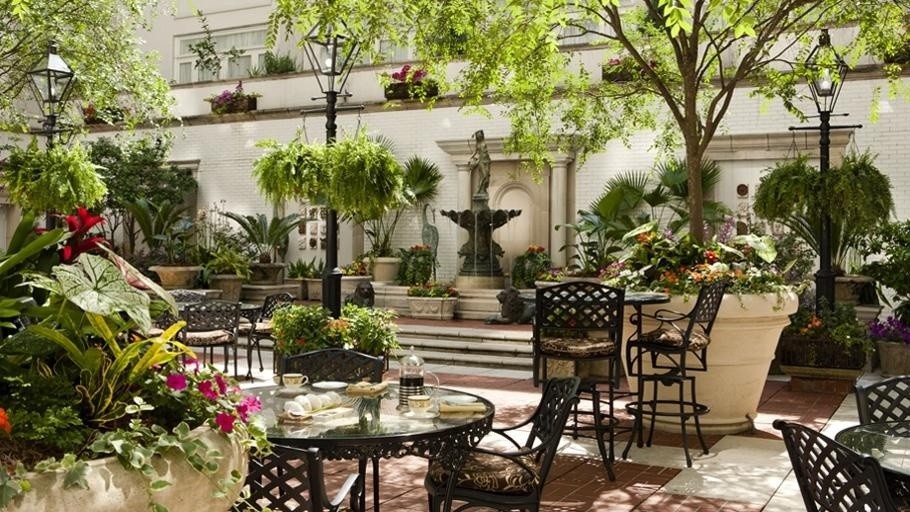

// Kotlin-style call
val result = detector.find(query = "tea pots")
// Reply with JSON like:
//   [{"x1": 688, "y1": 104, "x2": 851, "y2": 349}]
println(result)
[{"x1": 396, "y1": 346, "x2": 439, "y2": 410}]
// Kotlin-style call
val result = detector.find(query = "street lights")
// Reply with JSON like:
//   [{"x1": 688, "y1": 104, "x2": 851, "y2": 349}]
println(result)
[
  {"x1": 803, "y1": 21, "x2": 851, "y2": 310},
  {"x1": 304, "y1": 14, "x2": 362, "y2": 316},
  {"x1": 26, "y1": 39, "x2": 73, "y2": 234}
]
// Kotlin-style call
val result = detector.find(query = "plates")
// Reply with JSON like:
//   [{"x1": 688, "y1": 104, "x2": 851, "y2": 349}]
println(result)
[
  {"x1": 277, "y1": 389, "x2": 310, "y2": 395},
  {"x1": 401, "y1": 411, "x2": 441, "y2": 419},
  {"x1": 441, "y1": 395, "x2": 478, "y2": 403},
  {"x1": 313, "y1": 381, "x2": 348, "y2": 389}
]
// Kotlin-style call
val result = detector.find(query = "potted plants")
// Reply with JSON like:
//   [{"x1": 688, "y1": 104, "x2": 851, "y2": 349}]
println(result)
[
  {"x1": 756, "y1": 147, "x2": 910, "y2": 308},
  {"x1": 345, "y1": 136, "x2": 443, "y2": 282},
  {"x1": 117, "y1": 195, "x2": 327, "y2": 302}
]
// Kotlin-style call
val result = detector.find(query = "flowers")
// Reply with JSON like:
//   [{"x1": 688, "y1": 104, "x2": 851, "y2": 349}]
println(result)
[
  {"x1": 380, "y1": 64, "x2": 432, "y2": 82},
  {"x1": 606, "y1": 56, "x2": 659, "y2": 70},
  {"x1": 82, "y1": 101, "x2": 132, "y2": 116},
  {"x1": 867, "y1": 317, "x2": 910, "y2": 344},
  {"x1": 780, "y1": 297, "x2": 864, "y2": 351},
  {"x1": 204, "y1": 80, "x2": 263, "y2": 114}
]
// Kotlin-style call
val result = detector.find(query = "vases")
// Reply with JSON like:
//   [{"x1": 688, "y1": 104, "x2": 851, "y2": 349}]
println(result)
[
  {"x1": 874, "y1": 340, "x2": 910, "y2": 377},
  {"x1": 210, "y1": 97, "x2": 258, "y2": 112},
  {"x1": 782, "y1": 332, "x2": 865, "y2": 370},
  {"x1": 601, "y1": 68, "x2": 657, "y2": 83},
  {"x1": 84, "y1": 113, "x2": 123, "y2": 124},
  {"x1": 384, "y1": 81, "x2": 438, "y2": 99}
]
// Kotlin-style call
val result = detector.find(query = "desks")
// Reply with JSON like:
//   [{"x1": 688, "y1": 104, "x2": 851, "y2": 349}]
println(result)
[{"x1": 835, "y1": 420, "x2": 910, "y2": 512}]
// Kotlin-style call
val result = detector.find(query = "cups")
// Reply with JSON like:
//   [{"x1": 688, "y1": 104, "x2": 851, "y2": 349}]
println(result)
[
  {"x1": 408, "y1": 396, "x2": 434, "y2": 415},
  {"x1": 283, "y1": 374, "x2": 308, "y2": 389}
]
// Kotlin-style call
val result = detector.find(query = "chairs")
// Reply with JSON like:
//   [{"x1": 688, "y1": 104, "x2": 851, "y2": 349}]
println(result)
[
  {"x1": 855, "y1": 375, "x2": 909, "y2": 436},
  {"x1": 773, "y1": 420, "x2": 898, "y2": 511}
]
